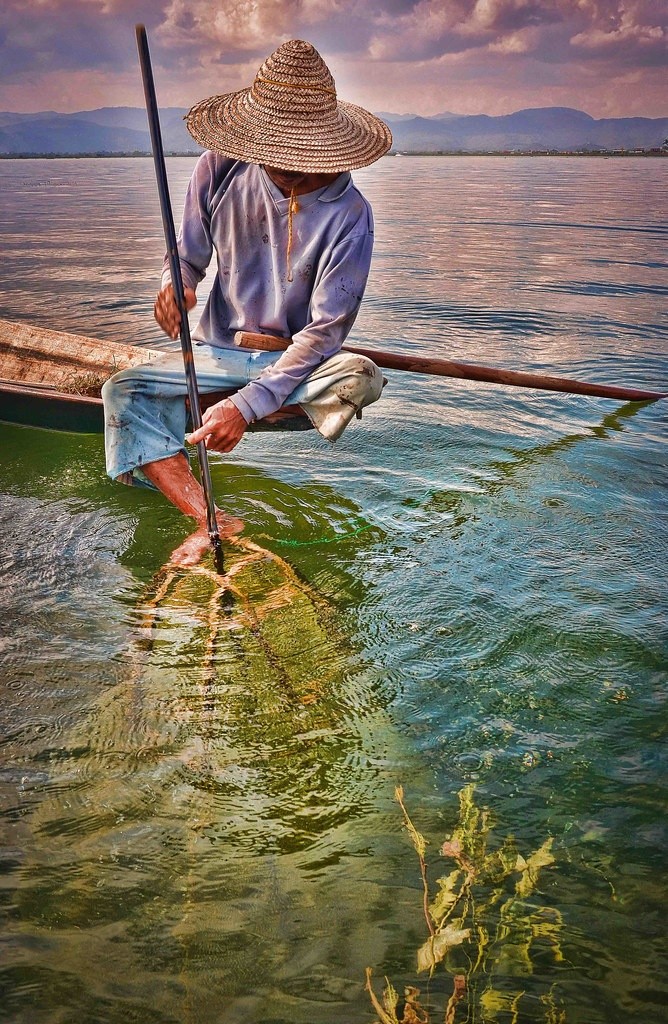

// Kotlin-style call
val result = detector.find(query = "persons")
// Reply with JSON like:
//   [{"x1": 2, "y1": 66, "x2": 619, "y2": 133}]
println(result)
[{"x1": 100, "y1": 39, "x2": 393, "y2": 567}]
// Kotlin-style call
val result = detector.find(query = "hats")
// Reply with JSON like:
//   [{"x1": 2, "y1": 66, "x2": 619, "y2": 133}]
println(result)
[{"x1": 181, "y1": 39, "x2": 393, "y2": 174}]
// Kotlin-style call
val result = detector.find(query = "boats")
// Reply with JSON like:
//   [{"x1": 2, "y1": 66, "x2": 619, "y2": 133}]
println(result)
[{"x1": 0, "y1": 318, "x2": 320, "y2": 433}]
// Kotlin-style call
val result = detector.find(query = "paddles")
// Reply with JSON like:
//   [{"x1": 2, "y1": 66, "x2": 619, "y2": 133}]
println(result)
[{"x1": 233, "y1": 328, "x2": 668, "y2": 404}]
